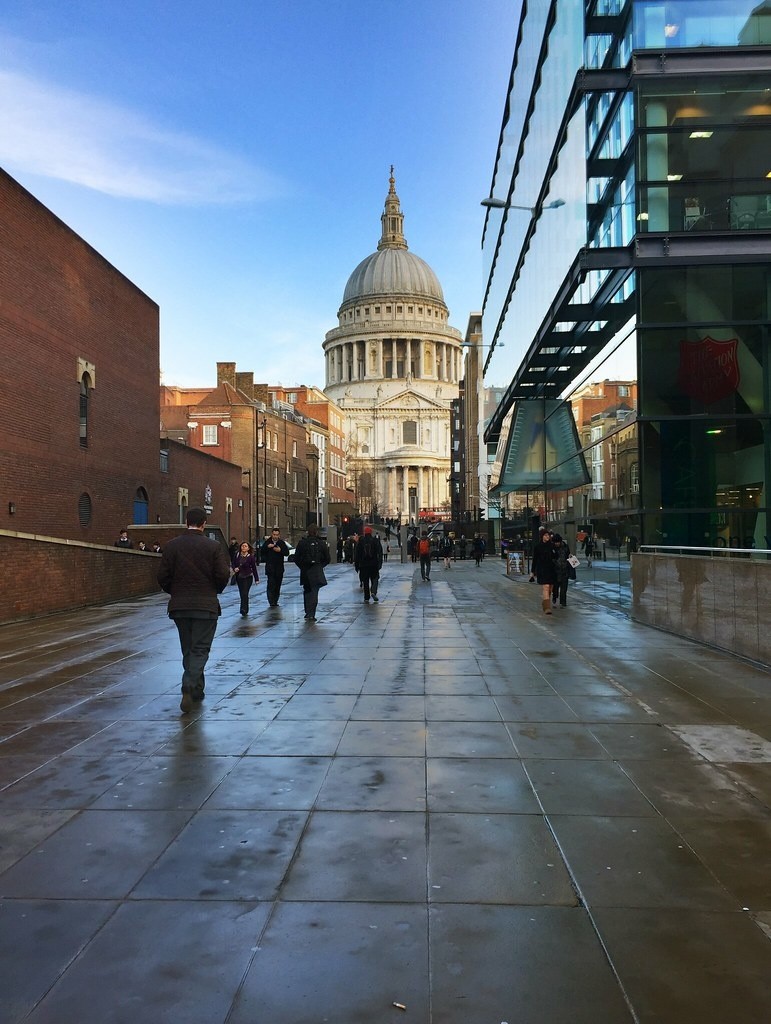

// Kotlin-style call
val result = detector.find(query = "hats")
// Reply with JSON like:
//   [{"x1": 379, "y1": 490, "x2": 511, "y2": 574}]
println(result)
[
  {"x1": 539, "y1": 526, "x2": 549, "y2": 538},
  {"x1": 552, "y1": 534, "x2": 563, "y2": 542},
  {"x1": 365, "y1": 527, "x2": 372, "y2": 533}
]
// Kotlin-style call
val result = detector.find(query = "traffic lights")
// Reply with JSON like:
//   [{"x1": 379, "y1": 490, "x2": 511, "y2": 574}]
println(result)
[
  {"x1": 343, "y1": 517, "x2": 348, "y2": 526},
  {"x1": 355, "y1": 513, "x2": 362, "y2": 527},
  {"x1": 477, "y1": 507, "x2": 485, "y2": 524},
  {"x1": 333, "y1": 514, "x2": 341, "y2": 527}
]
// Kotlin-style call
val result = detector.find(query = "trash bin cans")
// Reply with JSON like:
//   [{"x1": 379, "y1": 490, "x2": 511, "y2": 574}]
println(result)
[{"x1": 501, "y1": 539, "x2": 510, "y2": 559}]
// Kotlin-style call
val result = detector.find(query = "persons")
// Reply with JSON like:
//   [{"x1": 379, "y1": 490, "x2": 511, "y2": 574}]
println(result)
[
  {"x1": 229, "y1": 537, "x2": 239, "y2": 585},
  {"x1": 153, "y1": 542, "x2": 163, "y2": 553},
  {"x1": 531, "y1": 526, "x2": 558, "y2": 614},
  {"x1": 678, "y1": 528, "x2": 714, "y2": 611},
  {"x1": 260, "y1": 528, "x2": 289, "y2": 606},
  {"x1": 416, "y1": 531, "x2": 431, "y2": 582},
  {"x1": 437, "y1": 531, "x2": 487, "y2": 570},
  {"x1": 139, "y1": 540, "x2": 151, "y2": 552},
  {"x1": 156, "y1": 507, "x2": 230, "y2": 713},
  {"x1": 232, "y1": 542, "x2": 259, "y2": 616},
  {"x1": 375, "y1": 517, "x2": 418, "y2": 563},
  {"x1": 551, "y1": 535, "x2": 572, "y2": 608},
  {"x1": 114, "y1": 529, "x2": 133, "y2": 549},
  {"x1": 293, "y1": 524, "x2": 331, "y2": 621},
  {"x1": 354, "y1": 527, "x2": 383, "y2": 602},
  {"x1": 581, "y1": 531, "x2": 594, "y2": 568},
  {"x1": 336, "y1": 533, "x2": 365, "y2": 587}
]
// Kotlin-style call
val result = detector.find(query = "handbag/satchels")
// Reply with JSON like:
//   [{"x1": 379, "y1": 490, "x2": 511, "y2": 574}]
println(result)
[
  {"x1": 231, "y1": 574, "x2": 237, "y2": 585},
  {"x1": 568, "y1": 554, "x2": 580, "y2": 569}
]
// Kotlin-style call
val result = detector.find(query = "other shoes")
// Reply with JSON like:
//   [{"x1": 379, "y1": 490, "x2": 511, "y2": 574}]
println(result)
[
  {"x1": 422, "y1": 578, "x2": 425, "y2": 581},
  {"x1": 199, "y1": 692, "x2": 206, "y2": 700},
  {"x1": 365, "y1": 595, "x2": 370, "y2": 601},
  {"x1": 304, "y1": 612, "x2": 311, "y2": 618},
  {"x1": 240, "y1": 609, "x2": 246, "y2": 616},
  {"x1": 309, "y1": 616, "x2": 317, "y2": 621},
  {"x1": 425, "y1": 576, "x2": 430, "y2": 580},
  {"x1": 560, "y1": 601, "x2": 567, "y2": 606},
  {"x1": 371, "y1": 593, "x2": 379, "y2": 602},
  {"x1": 180, "y1": 683, "x2": 193, "y2": 713},
  {"x1": 552, "y1": 596, "x2": 556, "y2": 603}
]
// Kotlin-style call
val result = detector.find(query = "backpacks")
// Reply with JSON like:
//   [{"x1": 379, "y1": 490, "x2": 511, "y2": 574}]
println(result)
[
  {"x1": 302, "y1": 538, "x2": 322, "y2": 566},
  {"x1": 420, "y1": 538, "x2": 429, "y2": 556}
]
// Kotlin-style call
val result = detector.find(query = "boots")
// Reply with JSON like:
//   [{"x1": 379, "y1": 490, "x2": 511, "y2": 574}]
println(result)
[{"x1": 543, "y1": 599, "x2": 552, "y2": 614}]
